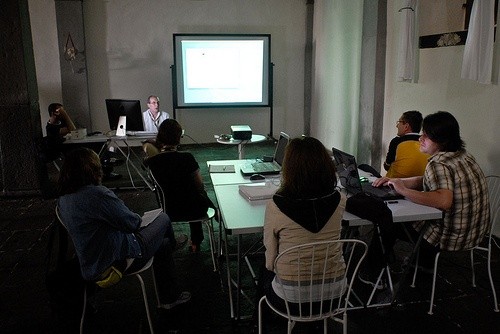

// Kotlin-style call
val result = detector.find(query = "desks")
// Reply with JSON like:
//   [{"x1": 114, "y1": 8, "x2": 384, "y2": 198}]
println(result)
[
  {"x1": 207, "y1": 160, "x2": 444, "y2": 320},
  {"x1": 64, "y1": 130, "x2": 185, "y2": 189},
  {"x1": 217, "y1": 134, "x2": 266, "y2": 159}
]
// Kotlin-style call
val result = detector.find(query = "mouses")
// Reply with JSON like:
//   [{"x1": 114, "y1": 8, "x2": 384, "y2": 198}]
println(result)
[{"x1": 250, "y1": 174, "x2": 265, "y2": 180}]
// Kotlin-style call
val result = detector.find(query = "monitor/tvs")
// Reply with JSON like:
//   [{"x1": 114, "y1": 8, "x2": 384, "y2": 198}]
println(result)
[{"x1": 105, "y1": 99, "x2": 144, "y2": 137}]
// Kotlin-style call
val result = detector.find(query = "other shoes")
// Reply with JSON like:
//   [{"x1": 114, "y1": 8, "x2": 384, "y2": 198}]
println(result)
[
  {"x1": 174, "y1": 234, "x2": 189, "y2": 252},
  {"x1": 160, "y1": 291, "x2": 191, "y2": 310}
]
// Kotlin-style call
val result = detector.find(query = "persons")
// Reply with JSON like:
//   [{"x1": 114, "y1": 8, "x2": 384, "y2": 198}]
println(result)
[
  {"x1": 57, "y1": 147, "x2": 191, "y2": 310},
  {"x1": 142, "y1": 95, "x2": 170, "y2": 132},
  {"x1": 384, "y1": 110, "x2": 431, "y2": 179},
  {"x1": 46, "y1": 103, "x2": 77, "y2": 148},
  {"x1": 358, "y1": 111, "x2": 491, "y2": 304},
  {"x1": 255, "y1": 137, "x2": 348, "y2": 334},
  {"x1": 142, "y1": 119, "x2": 218, "y2": 257}
]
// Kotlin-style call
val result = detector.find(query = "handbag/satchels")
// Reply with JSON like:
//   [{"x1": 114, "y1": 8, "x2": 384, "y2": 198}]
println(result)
[{"x1": 346, "y1": 194, "x2": 396, "y2": 246}]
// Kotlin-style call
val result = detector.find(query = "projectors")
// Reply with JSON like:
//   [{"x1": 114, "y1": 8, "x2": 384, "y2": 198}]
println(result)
[{"x1": 230, "y1": 124, "x2": 252, "y2": 140}]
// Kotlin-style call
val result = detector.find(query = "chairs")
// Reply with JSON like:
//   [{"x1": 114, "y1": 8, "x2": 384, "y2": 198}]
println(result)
[
  {"x1": 258, "y1": 239, "x2": 368, "y2": 334},
  {"x1": 150, "y1": 169, "x2": 216, "y2": 272},
  {"x1": 411, "y1": 175, "x2": 500, "y2": 315},
  {"x1": 55, "y1": 206, "x2": 161, "y2": 334}
]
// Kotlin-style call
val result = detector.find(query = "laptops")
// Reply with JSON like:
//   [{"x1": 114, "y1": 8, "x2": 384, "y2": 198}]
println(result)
[
  {"x1": 240, "y1": 131, "x2": 290, "y2": 175},
  {"x1": 332, "y1": 147, "x2": 406, "y2": 200}
]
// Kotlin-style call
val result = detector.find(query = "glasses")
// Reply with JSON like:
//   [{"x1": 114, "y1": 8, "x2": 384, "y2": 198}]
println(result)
[{"x1": 396, "y1": 121, "x2": 404, "y2": 124}]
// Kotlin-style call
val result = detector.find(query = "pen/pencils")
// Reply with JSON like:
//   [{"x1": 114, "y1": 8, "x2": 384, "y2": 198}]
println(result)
[{"x1": 223, "y1": 166, "x2": 226, "y2": 170}]
